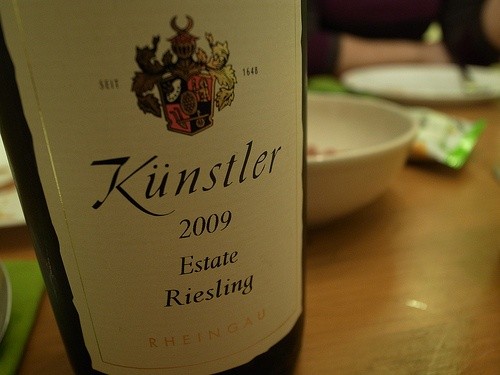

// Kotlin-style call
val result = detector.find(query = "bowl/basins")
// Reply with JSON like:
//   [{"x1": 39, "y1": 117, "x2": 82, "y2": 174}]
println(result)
[{"x1": 308, "y1": 94, "x2": 417, "y2": 226}]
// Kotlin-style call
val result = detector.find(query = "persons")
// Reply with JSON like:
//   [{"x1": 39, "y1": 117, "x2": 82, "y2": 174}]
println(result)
[{"x1": 305, "y1": 0, "x2": 499, "y2": 78}]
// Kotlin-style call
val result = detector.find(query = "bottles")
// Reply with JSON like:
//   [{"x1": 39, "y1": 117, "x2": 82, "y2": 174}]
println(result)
[{"x1": 0, "y1": 0, "x2": 305, "y2": 373}]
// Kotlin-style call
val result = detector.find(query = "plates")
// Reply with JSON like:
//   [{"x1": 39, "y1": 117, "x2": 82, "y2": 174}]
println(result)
[{"x1": 343, "y1": 62, "x2": 499, "y2": 101}]
